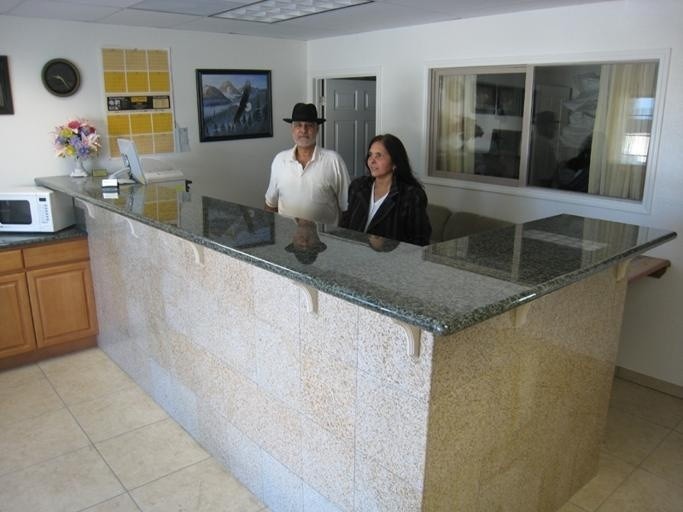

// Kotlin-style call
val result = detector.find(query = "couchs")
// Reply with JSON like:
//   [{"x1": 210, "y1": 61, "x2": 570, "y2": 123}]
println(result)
[{"x1": 425, "y1": 202, "x2": 513, "y2": 254}]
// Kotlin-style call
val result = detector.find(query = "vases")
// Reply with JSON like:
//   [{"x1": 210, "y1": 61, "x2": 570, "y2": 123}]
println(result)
[{"x1": 71, "y1": 158, "x2": 90, "y2": 177}]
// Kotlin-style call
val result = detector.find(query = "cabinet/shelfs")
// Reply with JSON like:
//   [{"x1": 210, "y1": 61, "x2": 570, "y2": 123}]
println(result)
[
  {"x1": 22, "y1": 239, "x2": 100, "y2": 349},
  {"x1": 0, "y1": 249, "x2": 36, "y2": 359}
]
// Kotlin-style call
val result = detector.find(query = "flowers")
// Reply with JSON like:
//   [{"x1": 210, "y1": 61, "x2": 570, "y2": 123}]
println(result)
[{"x1": 49, "y1": 119, "x2": 103, "y2": 161}]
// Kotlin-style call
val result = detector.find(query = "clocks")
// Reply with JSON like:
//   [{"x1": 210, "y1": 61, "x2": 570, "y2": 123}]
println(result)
[{"x1": 41, "y1": 58, "x2": 80, "y2": 97}]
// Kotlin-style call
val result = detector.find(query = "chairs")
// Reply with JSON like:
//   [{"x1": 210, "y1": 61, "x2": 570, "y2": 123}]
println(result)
[{"x1": 477, "y1": 150, "x2": 520, "y2": 180}]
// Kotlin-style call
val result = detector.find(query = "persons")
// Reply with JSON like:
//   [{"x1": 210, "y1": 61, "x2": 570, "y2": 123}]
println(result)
[
  {"x1": 367, "y1": 234, "x2": 399, "y2": 253},
  {"x1": 285, "y1": 218, "x2": 327, "y2": 264},
  {"x1": 524, "y1": 111, "x2": 560, "y2": 188},
  {"x1": 265, "y1": 104, "x2": 350, "y2": 225},
  {"x1": 341, "y1": 134, "x2": 432, "y2": 247}
]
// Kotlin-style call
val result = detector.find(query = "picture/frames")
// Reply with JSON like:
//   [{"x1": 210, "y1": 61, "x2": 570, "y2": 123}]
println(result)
[
  {"x1": 476, "y1": 82, "x2": 496, "y2": 114},
  {"x1": 497, "y1": 86, "x2": 523, "y2": 115},
  {"x1": 1, "y1": 56, "x2": 14, "y2": 114},
  {"x1": 202, "y1": 193, "x2": 275, "y2": 248},
  {"x1": 196, "y1": 68, "x2": 273, "y2": 143}
]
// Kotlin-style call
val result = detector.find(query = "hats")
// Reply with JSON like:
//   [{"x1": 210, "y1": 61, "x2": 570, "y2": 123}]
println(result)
[{"x1": 283, "y1": 102, "x2": 326, "y2": 125}]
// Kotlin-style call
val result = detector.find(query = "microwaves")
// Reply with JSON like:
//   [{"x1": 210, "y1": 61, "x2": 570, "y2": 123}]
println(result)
[{"x1": 0, "y1": 186, "x2": 76, "y2": 233}]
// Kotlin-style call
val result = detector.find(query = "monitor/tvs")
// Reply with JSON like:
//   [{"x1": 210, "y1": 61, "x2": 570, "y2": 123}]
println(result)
[
  {"x1": 627, "y1": 96, "x2": 655, "y2": 119},
  {"x1": 616, "y1": 131, "x2": 650, "y2": 165},
  {"x1": 108, "y1": 138, "x2": 146, "y2": 186}
]
